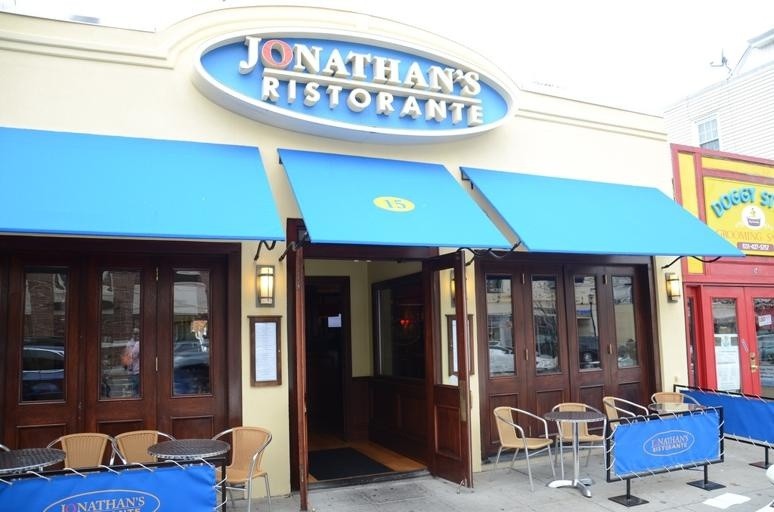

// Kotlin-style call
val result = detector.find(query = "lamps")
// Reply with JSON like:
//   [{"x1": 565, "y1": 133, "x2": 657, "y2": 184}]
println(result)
[
  {"x1": 255, "y1": 265, "x2": 276, "y2": 308},
  {"x1": 665, "y1": 271, "x2": 681, "y2": 302}
]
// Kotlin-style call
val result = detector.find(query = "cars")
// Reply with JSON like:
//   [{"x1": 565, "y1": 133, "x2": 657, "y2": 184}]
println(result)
[
  {"x1": 487, "y1": 336, "x2": 601, "y2": 375},
  {"x1": 20, "y1": 340, "x2": 114, "y2": 402},
  {"x1": 174, "y1": 338, "x2": 213, "y2": 374}
]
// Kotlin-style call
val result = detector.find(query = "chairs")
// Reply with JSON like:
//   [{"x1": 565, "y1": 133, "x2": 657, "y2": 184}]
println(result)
[
  {"x1": 40, "y1": 426, "x2": 273, "y2": 511},
  {"x1": 489, "y1": 392, "x2": 706, "y2": 498}
]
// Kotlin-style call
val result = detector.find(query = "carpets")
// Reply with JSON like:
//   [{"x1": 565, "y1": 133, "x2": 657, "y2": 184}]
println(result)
[{"x1": 306, "y1": 446, "x2": 395, "y2": 480}]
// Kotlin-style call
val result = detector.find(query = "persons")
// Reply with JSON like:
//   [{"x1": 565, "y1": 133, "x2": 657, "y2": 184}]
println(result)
[{"x1": 122, "y1": 328, "x2": 140, "y2": 396}]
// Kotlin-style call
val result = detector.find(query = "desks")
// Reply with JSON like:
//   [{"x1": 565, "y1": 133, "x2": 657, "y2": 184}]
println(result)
[{"x1": 0, "y1": 447, "x2": 67, "y2": 481}]
